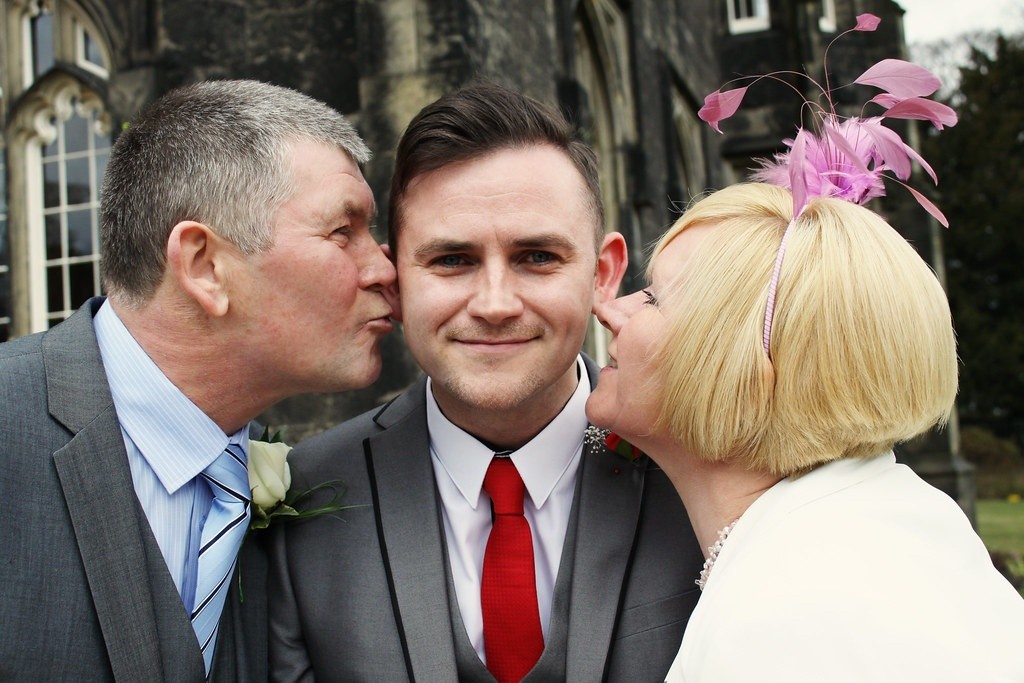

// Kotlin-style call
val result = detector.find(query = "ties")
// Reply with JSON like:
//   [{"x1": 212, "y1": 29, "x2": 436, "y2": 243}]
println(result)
[
  {"x1": 483, "y1": 458, "x2": 548, "y2": 683},
  {"x1": 190, "y1": 444, "x2": 251, "y2": 678}
]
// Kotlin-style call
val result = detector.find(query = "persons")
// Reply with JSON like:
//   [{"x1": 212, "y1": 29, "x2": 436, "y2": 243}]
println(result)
[
  {"x1": 204, "y1": 86, "x2": 709, "y2": 682},
  {"x1": 585, "y1": 183, "x2": 1024, "y2": 683},
  {"x1": 0, "y1": 79, "x2": 398, "y2": 683}
]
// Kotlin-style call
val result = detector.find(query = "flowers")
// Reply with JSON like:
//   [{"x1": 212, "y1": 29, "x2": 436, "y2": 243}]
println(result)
[
  {"x1": 697, "y1": 14, "x2": 962, "y2": 231},
  {"x1": 235, "y1": 424, "x2": 371, "y2": 603},
  {"x1": 582, "y1": 424, "x2": 646, "y2": 467}
]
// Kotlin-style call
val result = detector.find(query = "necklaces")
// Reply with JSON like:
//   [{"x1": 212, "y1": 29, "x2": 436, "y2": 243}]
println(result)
[{"x1": 694, "y1": 517, "x2": 740, "y2": 592}]
